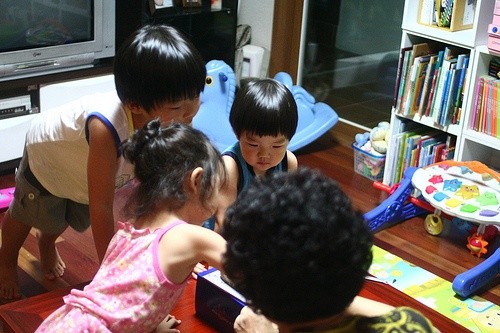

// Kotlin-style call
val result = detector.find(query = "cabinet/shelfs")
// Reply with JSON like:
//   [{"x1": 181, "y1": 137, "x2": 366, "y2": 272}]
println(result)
[{"x1": 373, "y1": 1, "x2": 500, "y2": 232}]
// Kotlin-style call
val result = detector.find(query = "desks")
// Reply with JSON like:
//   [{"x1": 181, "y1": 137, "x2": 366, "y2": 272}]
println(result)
[{"x1": 0, "y1": 229, "x2": 476, "y2": 333}]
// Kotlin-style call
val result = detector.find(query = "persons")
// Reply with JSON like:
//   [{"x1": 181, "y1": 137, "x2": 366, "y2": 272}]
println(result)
[
  {"x1": 221, "y1": 166, "x2": 444, "y2": 333},
  {"x1": 33, "y1": 116, "x2": 230, "y2": 333},
  {"x1": 0, "y1": 23, "x2": 207, "y2": 301},
  {"x1": 202, "y1": 76, "x2": 299, "y2": 235}
]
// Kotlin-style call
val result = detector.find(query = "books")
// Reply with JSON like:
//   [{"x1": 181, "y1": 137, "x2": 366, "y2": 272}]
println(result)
[
  {"x1": 487, "y1": 0, "x2": 500, "y2": 54},
  {"x1": 393, "y1": 42, "x2": 471, "y2": 127},
  {"x1": 388, "y1": 118, "x2": 456, "y2": 205},
  {"x1": 412, "y1": 0, "x2": 479, "y2": 31},
  {"x1": 471, "y1": 74, "x2": 500, "y2": 138}
]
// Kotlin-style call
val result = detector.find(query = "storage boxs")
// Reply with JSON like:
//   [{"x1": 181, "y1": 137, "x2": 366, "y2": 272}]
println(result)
[{"x1": 351, "y1": 144, "x2": 387, "y2": 182}]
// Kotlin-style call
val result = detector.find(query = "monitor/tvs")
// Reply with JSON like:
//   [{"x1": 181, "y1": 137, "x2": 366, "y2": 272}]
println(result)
[{"x1": 0, "y1": 0, "x2": 116, "y2": 81}]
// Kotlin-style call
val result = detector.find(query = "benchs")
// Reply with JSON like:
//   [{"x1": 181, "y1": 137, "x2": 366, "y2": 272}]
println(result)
[{"x1": 195, "y1": 60, "x2": 338, "y2": 157}]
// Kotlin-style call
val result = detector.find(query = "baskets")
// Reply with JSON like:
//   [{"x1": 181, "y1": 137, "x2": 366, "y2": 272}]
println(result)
[{"x1": 352, "y1": 143, "x2": 386, "y2": 181}]
